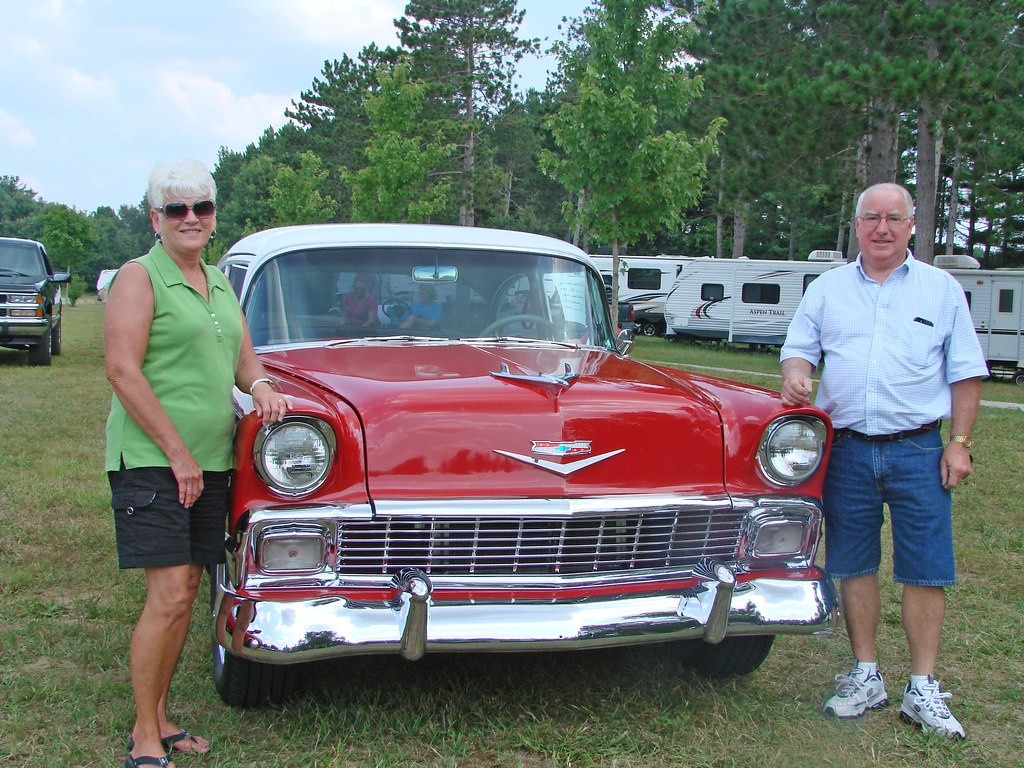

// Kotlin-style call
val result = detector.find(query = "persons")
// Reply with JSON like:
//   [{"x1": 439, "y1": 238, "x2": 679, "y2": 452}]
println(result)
[
  {"x1": 105, "y1": 161, "x2": 292, "y2": 768},
  {"x1": 401, "y1": 284, "x2": 443, "y2": 332},
  {"x1": 342, "y1": 276, "x2": 382, "y2": 330},
  {"x1": 779, "y1": 183, "x2": 989, "y2": 742}
]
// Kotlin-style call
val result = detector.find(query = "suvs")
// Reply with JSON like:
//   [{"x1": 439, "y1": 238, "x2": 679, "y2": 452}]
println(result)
[{"x1": 0, "y1": 237, "x2": 71, "y2": 366}]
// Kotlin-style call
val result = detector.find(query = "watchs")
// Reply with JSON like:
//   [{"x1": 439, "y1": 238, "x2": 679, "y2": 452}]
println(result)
[
  {"x1": 250, "y1": 378, "x2": 278, "y2": 395},
  {"x1": 950, "y1": 435, "x2": 972, "y2": 450}
]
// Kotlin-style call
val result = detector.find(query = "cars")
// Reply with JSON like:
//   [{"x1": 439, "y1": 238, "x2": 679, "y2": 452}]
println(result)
[{"x1": 209, "y1": 222, "x2": 838, "y2": 713}]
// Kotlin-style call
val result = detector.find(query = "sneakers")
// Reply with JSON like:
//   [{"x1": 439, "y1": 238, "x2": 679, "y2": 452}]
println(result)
[
  {"x1": 822, "y1": 658, "x2": 890, "y2": 719},
  {"x1": 899, "y1": 675, "x2": 966, "y2": 741}
]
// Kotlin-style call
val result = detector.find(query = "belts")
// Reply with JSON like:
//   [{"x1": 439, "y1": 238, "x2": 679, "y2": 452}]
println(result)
[{"x1": 835, "y1": 420, "x2": 938, "y2": 442}]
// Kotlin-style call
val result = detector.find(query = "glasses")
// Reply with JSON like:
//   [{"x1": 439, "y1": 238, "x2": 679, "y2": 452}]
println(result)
[
  {"x1": 858, "y1": 214, "x2": 911, "y2": 226},
  {"x1": 154, "y1": 198, "x2": 217, "y2": 221}
]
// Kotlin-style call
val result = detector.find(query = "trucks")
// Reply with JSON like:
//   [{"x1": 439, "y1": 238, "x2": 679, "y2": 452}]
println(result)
[
  {"x1": 587, "y1": 250, "x2": 1024, "y2": 386},
  {"x1": 336, "y1": 269, "x2": 485, "y2": 305}
]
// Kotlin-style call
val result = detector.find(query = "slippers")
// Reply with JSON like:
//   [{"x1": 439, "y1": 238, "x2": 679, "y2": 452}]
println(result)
[
  {"x1": 125, "y1": 753, "x2": 172, "y2": 768},
  {"x1": 126, "y1": 729, "x2": 198, "y2": 753}
]
